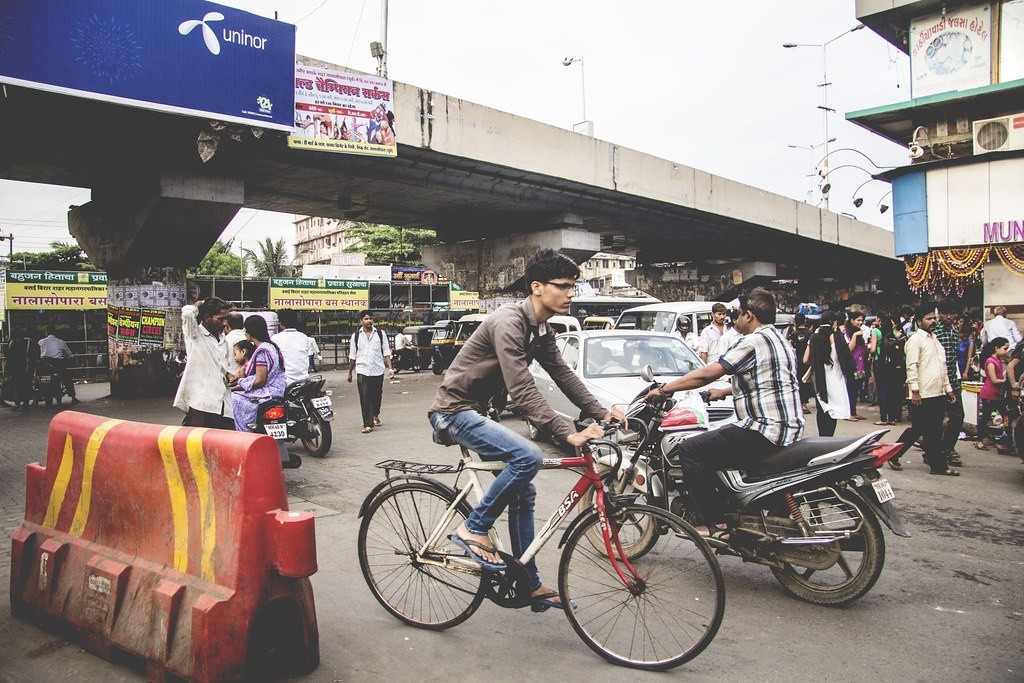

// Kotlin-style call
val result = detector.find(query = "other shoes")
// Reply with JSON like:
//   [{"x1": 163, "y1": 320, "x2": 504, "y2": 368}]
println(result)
[
  {"x1": 12, "y1": 403, "x2": 22, "y2": 409},
  {"x1": 950, "y1": 450, "x2": 961, "y2": 458},
  {"x1": 72, "y1": 398, "x2": 81, "y2": 404},
  {"x1": 976, "y1": 444, "x2": 989, "y2": 451},
  {"x1": 854, "y1": 414, "x2": 865, "y2": 419},
  {"x1": 362, "y1": 426, "x2": 372, "y2": 433},
  {"x1": 846, "y1": 416, "x2": 857, "y2": 421},
  {"x1": 31, "y1": 401, "x2": 38, "y2": 406},
  {"x1": 944, "y1": 454, "x2": 961, "y2": 465},
  {"x1": 373, "y1": 415, "x2": 382, "y2": 426},
  {"x1": 889, "y1": 420, "x2": 895, "y2": 425},
  {"x1": 873, "y1": 419, "x2": 887, "y2": 425}
]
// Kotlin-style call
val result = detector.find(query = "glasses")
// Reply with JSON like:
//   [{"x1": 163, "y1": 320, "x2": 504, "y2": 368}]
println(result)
[{"x1": 542, "y1": 279, "x2": 579, "y2": 291}]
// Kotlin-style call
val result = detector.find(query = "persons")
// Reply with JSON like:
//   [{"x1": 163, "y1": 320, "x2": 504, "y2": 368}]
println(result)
[
  {"x1": 789, "y1": 303, "x2": 1024, "y2": 439},
  {"x1": 640, "y1": 289, "x2": 805, "y2": 548},
  {"x1": 302, "y1": 110, "x2": 395, "y2": 145},
  {"x1": 429, "y1": 249, "x2": 628, "y2": 616},
  {"x1": 974, "y1": 337, "x2": 1009, "y2": 452},
  {"x1": 642, "y1": 277, "x2": 733, "y2": 302},
  {"x1": 888, "y1": 304, "x2": 964, "y2": 476},
  {"x1": 3, "y1": 323, "x2": 81, "y2": 408},
  {"x1": 348, "y1": 310, "x2": 395, "y2": 434},
  {"x1": 736, "y1": 281, "x2": 856, "y2": 314},
  {"x1": 173, "y1": 296, "x2": 286, "y2": 433},
  {"x1": 395, "y1": 328, "x2": 412, "y2": 364},
  {"x1": 270, "y1": 308, "x2": 320, "y2": 389}
]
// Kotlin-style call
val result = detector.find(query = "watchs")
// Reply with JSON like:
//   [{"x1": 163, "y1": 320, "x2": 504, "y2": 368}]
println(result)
[{"x1": 658, "y1": 382, "x2": 673, "y2": 400}]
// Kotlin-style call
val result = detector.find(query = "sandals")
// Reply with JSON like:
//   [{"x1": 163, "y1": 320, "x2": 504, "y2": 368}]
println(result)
[
  {"x1": 928, "y1": 466, "x2": 960, "y2": 476},
  {"x1": 675, "y1": 526, "x2": 731, "y2": 548},
  {"x1": 888, "y1": 459, "x2": 902, "y2": 470}
]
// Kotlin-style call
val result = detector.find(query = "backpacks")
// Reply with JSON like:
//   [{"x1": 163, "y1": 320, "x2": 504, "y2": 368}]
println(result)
[{"x1": 879, "y1": 328, "x2": 903, "y2": 363}]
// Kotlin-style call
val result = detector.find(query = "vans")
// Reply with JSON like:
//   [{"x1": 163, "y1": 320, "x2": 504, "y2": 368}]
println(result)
[{"x1": 615, "y1": 301, "x2": 821, "y2": 347}]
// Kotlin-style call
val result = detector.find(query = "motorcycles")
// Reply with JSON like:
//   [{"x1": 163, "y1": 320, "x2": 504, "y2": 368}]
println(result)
[
  {"x1": 578, "y1": 364, "x2": 912, "y2": 608},
  {"x1": 389, "y1": 344, "x2": 423, "y2": 374},
  {"x1": 246, "y1": 374, "x2": 335, "y2": 474},
  {"x1": 402, "y1": 316, "x2": 616, "y2": 375},
  {"x1": 35, "y1": 356, "x2": 72, "y2": 408}
]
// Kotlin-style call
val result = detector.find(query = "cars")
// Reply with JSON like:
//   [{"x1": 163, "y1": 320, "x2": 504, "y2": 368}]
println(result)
[{"x1": 529, "y1": 328, "x2": 734, "y2": 457}]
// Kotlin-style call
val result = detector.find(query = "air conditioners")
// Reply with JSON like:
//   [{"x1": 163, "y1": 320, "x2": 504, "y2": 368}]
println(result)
[{"x1": 973, "y1": 113, "x2": 1024, "y2": 157}]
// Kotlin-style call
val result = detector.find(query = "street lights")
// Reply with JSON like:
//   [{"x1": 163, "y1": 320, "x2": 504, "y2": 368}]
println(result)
[
  {"x1": 782, "y1": 23, "x2": 865, "y2": 210},
  {"x1": 562, "y1": 57, "x2": 585, "y2": 121}
]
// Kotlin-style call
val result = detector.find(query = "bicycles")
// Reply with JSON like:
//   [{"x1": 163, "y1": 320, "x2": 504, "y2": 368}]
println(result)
[{"x1": 357, "y1": 417, "x2": 726, "y2": 672}]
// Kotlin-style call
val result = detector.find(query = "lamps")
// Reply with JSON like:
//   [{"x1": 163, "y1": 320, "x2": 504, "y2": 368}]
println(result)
[
  {"x1": 907, "y1": 126, "x2": 945, "y2": 159},
  {"x1": 815, "y1": 148, "x2": 896, "y2": 214}
]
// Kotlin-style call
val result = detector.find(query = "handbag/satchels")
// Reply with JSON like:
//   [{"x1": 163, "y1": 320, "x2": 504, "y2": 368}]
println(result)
[{"x1": 658, "y1": 391, "x2": 708, "y2": 432}]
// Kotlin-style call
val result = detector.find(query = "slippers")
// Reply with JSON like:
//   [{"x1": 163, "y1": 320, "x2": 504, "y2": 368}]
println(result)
[
  {"x1": 524, "y1": 585, "x2": 578, "y2": 609},
  {"x1": 450, "y1": 532, "x2": 507, "y2": 571},
  {"x1": 802, "y1": 407, "x2": 811, "y2": 413}
]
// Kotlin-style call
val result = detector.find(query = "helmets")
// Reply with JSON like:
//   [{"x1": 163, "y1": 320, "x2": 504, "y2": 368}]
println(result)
[{"x1": 676, "y1": 316, "x2": 692, "y2": 332}]
